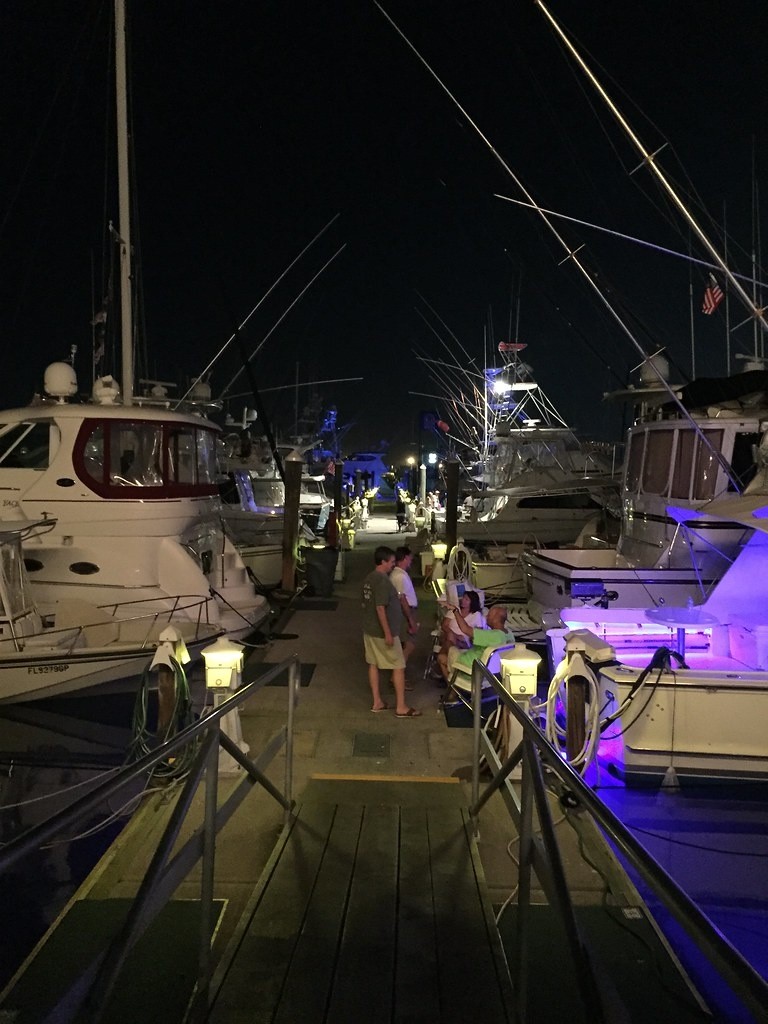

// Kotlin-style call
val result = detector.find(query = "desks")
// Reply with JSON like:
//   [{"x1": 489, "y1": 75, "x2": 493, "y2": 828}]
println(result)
[{"x1": 645, "y1": 606, "x2": 720, "y2": 668}]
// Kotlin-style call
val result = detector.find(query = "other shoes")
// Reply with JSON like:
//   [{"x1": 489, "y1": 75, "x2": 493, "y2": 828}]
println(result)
[
  {"x1": 390, "y1": 683, "x2": 413, "y2": 691},
  {"x1": 439, "y1": 694, "x2": 459, "y2": 704},
  {"x1": 390, "y1": 674, "x2": 411, "y2": 682}
]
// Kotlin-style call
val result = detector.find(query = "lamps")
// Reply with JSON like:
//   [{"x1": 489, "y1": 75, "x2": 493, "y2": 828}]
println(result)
[
  {"x1": 200, "y1": 637, "x2": 244, "y2": 688},
  {"x1": 498, "y1": 644, "x2": 542, "y2": 701}
]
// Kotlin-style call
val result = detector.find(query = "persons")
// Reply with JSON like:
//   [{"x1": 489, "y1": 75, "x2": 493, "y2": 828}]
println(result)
[
  {"x1": 442, "y1": 591, "x2": 483, "y2": 643},
  {"x1": 389, "y1": 546, "x2": 420, "y2": 690},
  {"x1": 439, "y1": 604, "x2": 515, "y2": 702},
  {"x1": 428, "y1": 491, "x2": 446, "y2": 508},
  {"x1": 396, "y1": 498, "x2": 406, "y2": 531},
  {"x1": 361, "y1": 546, "x2": 421, "y2": 717}
]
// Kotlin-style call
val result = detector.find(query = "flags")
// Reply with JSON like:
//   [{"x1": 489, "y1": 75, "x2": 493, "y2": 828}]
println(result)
[
  {"x1": 328, "y1": 461, "x2": 335, "y2": 475},
  {"x1": 702, "y1": 272, "x2": 724, "y2": 315}
]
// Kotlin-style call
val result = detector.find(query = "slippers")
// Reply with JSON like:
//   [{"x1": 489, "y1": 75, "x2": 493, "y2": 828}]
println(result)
[
  {"x1": 370, "y1": 703, "x2": 395, "y2": 712},
  {"x1": 395, "y1": 707, "x2": 422, "y2": 717}
]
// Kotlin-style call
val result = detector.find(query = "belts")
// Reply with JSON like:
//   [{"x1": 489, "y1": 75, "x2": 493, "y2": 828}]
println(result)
[{"x1": 409, "y1": 606, "x2": 418, "y2": 610}]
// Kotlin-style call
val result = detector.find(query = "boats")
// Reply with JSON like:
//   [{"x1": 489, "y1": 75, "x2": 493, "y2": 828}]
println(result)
[
  {"x1": 0, "y1": 0, "x2": 338, "y2": 720},
  {"x1": 385, "y1": 0, "x2": 768, "y2": 779}
]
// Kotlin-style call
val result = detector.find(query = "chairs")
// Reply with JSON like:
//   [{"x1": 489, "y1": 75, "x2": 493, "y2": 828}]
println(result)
[
  {"x1": 423, "y1": 630, "x2": 444, "y2": 679},
  {"x1": 14, "y1": 613, "x2": 86, "y2": 650},
  {"x1": 436, "y1": 641, "x2": 527, "y2": 720}
]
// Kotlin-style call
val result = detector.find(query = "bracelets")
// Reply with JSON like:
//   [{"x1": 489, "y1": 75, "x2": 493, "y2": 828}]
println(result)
[{"x1": 453, "y1": 608, "x2": 458, "y2": 613}]
